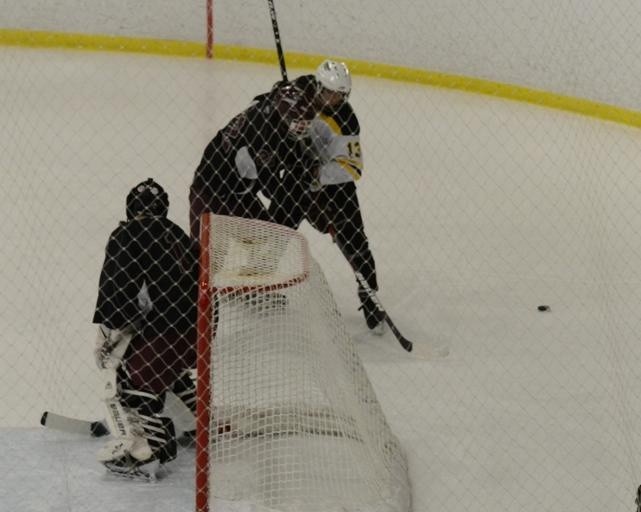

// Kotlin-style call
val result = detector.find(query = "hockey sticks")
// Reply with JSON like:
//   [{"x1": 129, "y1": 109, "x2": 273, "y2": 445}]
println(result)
[{"x1": 329, "y1": 225, "x2": 412, "y2": 352}]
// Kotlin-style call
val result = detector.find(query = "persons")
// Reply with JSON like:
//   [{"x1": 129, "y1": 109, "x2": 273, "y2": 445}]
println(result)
[
  {"x1": 188, "y1": 81, "x2": 334, "y2": 330},
  {"x1": 91, "y1": 177, "x2": 219, "y2": 478},
  {"x1": 291, "y1": 60, "x2": 386, "y2": 330}
]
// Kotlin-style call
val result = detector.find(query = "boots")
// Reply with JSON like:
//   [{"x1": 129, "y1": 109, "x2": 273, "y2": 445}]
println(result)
[
  {"x1": 357, "y1": 287, "x2": 388, "y2": 330},
  {"x1": 99, "y1": 449, "x2": 162, "y2": 477}
]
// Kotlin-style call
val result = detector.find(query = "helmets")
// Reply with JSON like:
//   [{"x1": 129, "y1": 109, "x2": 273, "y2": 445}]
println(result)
[
  {"x1": 125, "y1": 177, "x2": 171, "y2": 219},
  {"x1": 314, "y1": 59, "x2": 353, "y2": 97},
  {"x1": 270, "y1": 80, "x2": 317, "y2": 123}
]
